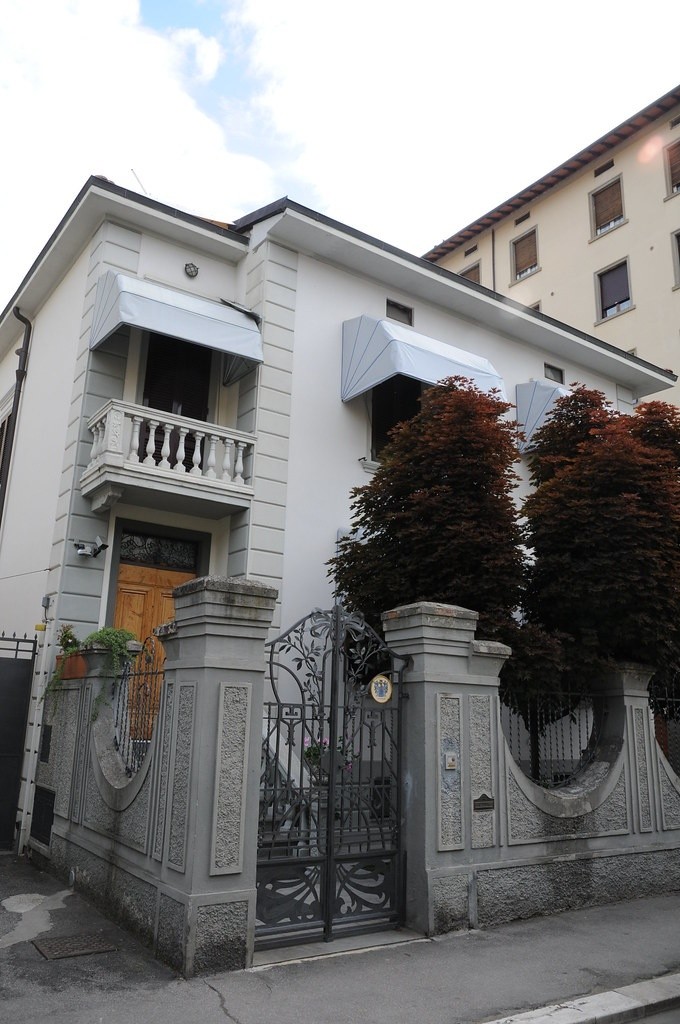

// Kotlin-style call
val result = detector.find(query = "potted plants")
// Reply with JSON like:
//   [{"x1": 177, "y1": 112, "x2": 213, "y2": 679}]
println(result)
[{"x1": 44, "y1": 625, "x2": 143, "y2": 720}]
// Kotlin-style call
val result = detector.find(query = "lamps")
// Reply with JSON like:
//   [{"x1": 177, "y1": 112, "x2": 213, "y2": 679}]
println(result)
[
  {"x1": 185, "y1": 262, "x2": 199, "y2": 276},
  {"x1": 77, "y1": 548, "x2": 93, "y2": 556}
]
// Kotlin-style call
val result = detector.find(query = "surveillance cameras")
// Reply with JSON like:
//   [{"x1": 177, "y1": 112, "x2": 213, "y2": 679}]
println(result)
[{"x1": 95, "y1": 535, "x2": 108, "y2": 550}]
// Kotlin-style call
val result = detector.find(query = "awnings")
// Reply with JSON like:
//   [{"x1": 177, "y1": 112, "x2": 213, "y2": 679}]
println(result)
[
  {"x1": 516, "y1": 381, "x2": 574, "y2": 453},
  {"x1": 341, "y1": 314, "x2": 507, "y2": 404},
  {"x1": 89, "y1": 270, "x2": 263, "y2": 385}
]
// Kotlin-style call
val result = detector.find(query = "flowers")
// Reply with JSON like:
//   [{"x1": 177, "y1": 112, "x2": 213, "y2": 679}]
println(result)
[{"x1": 300, "y1": 736, "x2": 353, "y2": 772}]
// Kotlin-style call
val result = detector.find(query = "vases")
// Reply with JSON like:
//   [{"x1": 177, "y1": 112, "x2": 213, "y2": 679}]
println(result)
[{"x1": 309, "y1": 764, "x2": 352, "y2": 785}]
